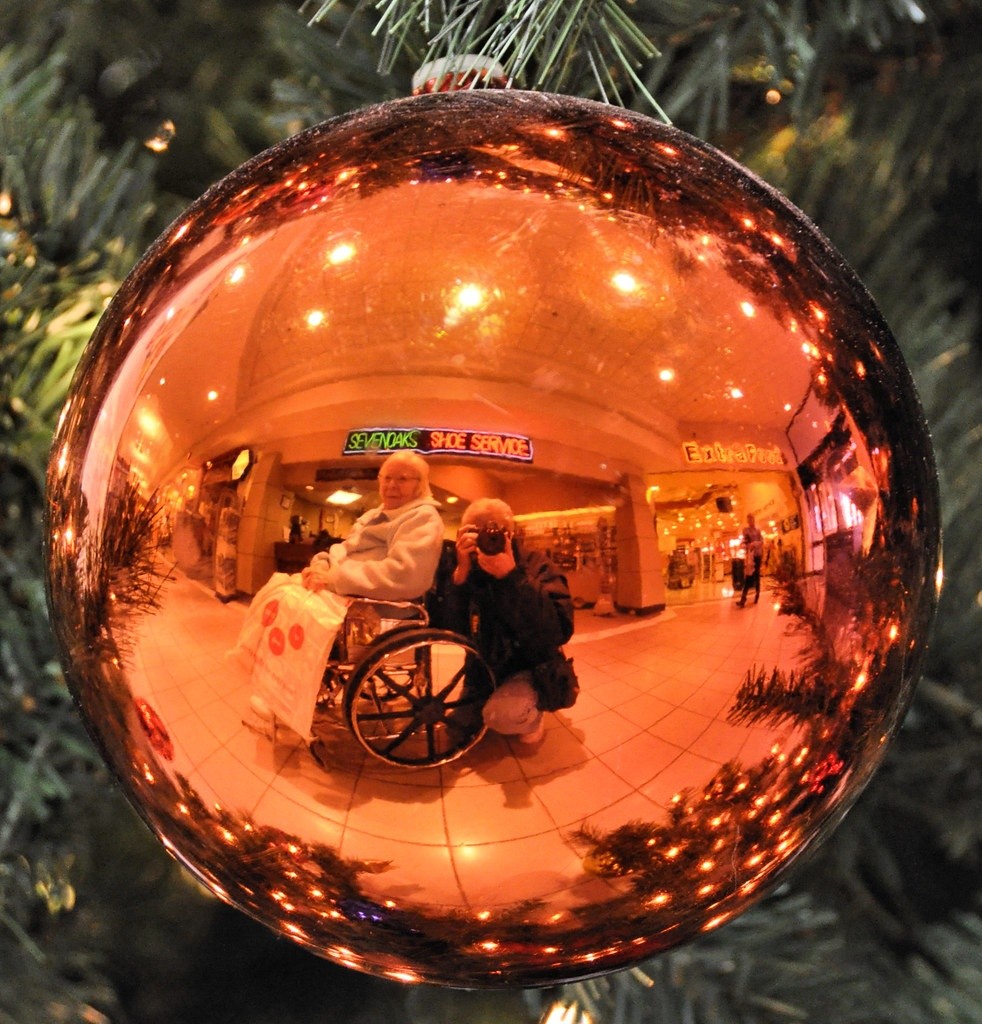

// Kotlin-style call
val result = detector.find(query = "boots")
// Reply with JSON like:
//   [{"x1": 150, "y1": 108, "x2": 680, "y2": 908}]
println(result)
[
  {"x1": 736, "y1": 596, "x2": 746, "y2": 608},
  {"x1": 754, "y1": 595, "x2": 759, "y2": 603}
]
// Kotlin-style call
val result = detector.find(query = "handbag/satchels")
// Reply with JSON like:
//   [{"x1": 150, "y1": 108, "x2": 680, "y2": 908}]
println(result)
[
  {"x1": 228, "y1": 572, "x2": 347, "y2": 745},
  {"x1": 534, "y1": 653, "x2": 580, "y2": 711}
]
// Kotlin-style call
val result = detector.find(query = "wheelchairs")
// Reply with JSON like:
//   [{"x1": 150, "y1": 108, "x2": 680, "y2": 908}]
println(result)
[{"x1": 307, "y1": 573, "x2": 500, "y2": 770}]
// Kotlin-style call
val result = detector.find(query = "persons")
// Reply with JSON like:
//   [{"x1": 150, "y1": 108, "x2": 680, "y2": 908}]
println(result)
[
  {"x1": 246, "y1": 449, "x2": 444, "y2": 727},
  {"x1": 734, "y1": 512, "x2": 764, "y2": 608},
  {"x1": 445, "y1": 498, "x2": 581, "y2": 744}
]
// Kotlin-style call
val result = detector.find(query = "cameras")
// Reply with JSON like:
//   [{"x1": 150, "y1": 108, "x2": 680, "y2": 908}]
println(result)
[{"x1": 470, "y1": 522, "x2": 507, "y2": 554}]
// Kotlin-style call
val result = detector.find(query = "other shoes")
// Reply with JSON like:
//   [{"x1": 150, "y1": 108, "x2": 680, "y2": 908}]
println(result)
[
  {"x1": 519, "y1": 711, "x2": 545, "y2": 744},
  {"x1": 277, "y1": 728, "x2": 314, "y2": 751},
  {"x1": 250, "y1": 696, "x2": 287, "y2": 727}
]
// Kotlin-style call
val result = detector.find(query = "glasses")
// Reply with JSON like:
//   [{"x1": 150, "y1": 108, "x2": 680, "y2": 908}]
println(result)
[{"x1": 378, "y1": 474, "x2": 421, "y2": 484}]
